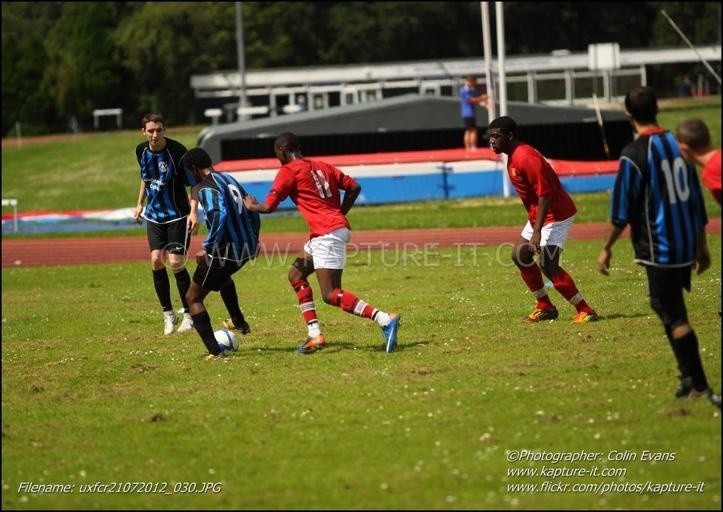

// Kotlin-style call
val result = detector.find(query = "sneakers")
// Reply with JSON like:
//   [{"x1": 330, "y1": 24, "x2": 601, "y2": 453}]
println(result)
[
  {"x1": 675, "y1": 375, "x2": 722, "y2": 408},
  {"x1": 163, "y1": 312, "x2": 179, "y2": 336},
  {"x1": 224, "y1": 319, "x2": 249, "y2": 335},
  {"x1": 299, "y1": 333, "x2": 325, "y2": 354},
  {"x1": 380, "y1": 313, "x2": 400, "y2": 353},
  {"x1": 571, "y1": 309, "x2": 597, "y2": 323},
  {"x1": 204, "y1": 352, "x2": 223, "y2": 361},
  {"x1": 178, "y1": 318, "x2": 194, "y2": 331},
  {"x1": 523, "y1": 306, "x2": 558, "y2": 323}
]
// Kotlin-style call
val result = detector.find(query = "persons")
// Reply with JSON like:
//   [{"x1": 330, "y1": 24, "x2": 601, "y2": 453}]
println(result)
[
  {"x1": 674, "y1": 119, "x2": 721, "y2": 205},
  {"x1": 489, "y1": 117, "x2": 599, "y2": 325},
  {"x1": 133, "y1": 111, "x2": 196, "y2": 336},
  {"x1": 243, "y1": 133, "x2": 401, "y2": 353},
  {"x1": 460, "y1": 72, "x2": 486, "y2": 153},
  {"x1": 595, "y1": 86, "x2": 712, "y2": 401},
  {"x1": 180, "y1": 147, "x2": 260, "y2": 360}
]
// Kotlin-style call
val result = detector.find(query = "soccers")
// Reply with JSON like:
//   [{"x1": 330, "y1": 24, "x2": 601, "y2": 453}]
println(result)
[{"x1": 212, "y1": 328, "x2": 238, "y2": 353}]
